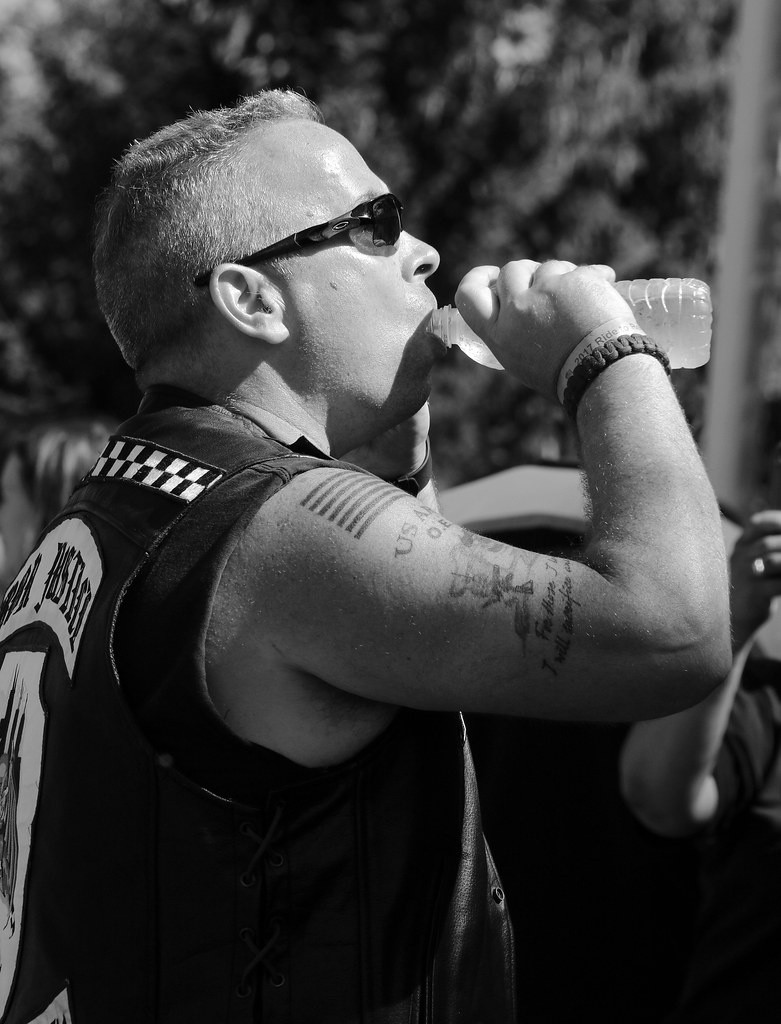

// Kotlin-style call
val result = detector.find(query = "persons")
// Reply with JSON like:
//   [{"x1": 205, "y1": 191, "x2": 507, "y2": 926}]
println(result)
[
  {"x1": 0, "y1": 90, "x2": 732, "y2": 1024},
  {"x1": 623, "y1": 387, "x2": 781, "y2": 1024}
]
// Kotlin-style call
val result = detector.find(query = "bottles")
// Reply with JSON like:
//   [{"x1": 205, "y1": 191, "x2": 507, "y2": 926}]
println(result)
[{"x1": 428, "y1": 278, "x2": 713, "y2": 371}]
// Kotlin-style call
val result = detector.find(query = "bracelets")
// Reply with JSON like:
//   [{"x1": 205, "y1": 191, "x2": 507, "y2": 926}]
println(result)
[{"x1": 552, "y1": 318, "x2": 674, "y2": 421}]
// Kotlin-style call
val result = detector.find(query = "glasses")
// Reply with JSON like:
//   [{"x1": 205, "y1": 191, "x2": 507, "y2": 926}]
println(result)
[{"x1": 193, "y1": 192, "x2": 403, "y2": 289}]
